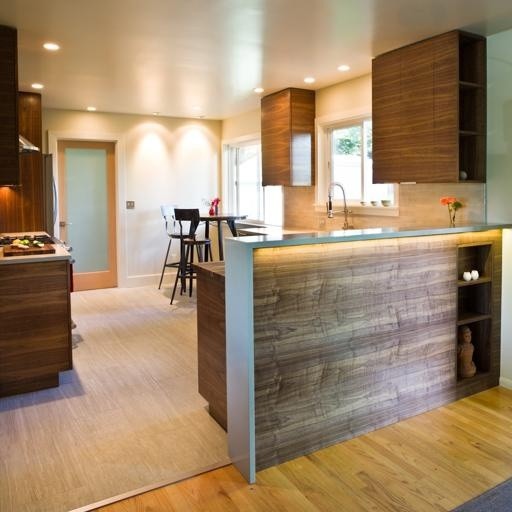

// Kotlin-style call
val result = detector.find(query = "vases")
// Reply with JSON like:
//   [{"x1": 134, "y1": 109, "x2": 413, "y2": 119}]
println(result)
[{"x1": 215, "y1": 204, "x2": 219, "y2": 216}]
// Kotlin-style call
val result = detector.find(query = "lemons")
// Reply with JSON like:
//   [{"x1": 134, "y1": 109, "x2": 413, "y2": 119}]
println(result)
[{"x1": 12, "y1": 238, "x2": 21, "y2": 248}]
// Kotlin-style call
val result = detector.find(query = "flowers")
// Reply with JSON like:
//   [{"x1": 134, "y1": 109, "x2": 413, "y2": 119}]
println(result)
[
  {"x1": 209, "y1": 198, "x2": 220, "y2": 216},
  {"x1": 440, "y1": 197, "x2": 463, "y2": 225}
]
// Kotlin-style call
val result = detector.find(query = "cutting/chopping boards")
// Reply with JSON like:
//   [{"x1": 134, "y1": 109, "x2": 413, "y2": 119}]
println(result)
[{"x1": 1, "y1": 243, "x2": 56, "y2": 256}]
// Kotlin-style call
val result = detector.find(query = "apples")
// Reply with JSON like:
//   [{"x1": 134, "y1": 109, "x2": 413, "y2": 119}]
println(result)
[
  {"x1": 32, "y1": 240, "x2": 45, "y2": 248},
  {"x1": 22, "y1": 239, "x2": 30, "y2": 247}
]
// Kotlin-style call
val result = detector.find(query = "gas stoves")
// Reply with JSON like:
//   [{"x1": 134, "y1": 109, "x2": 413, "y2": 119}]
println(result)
[{"x1": 0, "y1": 234, "x2": 55, "y2": 246}]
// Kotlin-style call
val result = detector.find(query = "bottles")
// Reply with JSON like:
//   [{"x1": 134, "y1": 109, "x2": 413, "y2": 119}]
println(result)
[{"x1": 209, "y1": 202, "x2": 215, "y2": 215}]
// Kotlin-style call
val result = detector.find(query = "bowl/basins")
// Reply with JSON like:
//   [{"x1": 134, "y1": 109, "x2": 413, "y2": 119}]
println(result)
[{"x1": 360, "y1": 200, "x2": 391, "y2": 207}]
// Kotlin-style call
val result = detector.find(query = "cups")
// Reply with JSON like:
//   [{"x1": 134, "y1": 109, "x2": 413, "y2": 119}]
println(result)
[{"x1": 463, "y1": 270, "x2": 480, "y2": 281}]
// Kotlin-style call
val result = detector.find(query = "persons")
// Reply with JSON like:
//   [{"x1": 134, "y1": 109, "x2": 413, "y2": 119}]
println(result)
[{"x1": 456, "y1": 326, "x2": 478, "y2": 378}]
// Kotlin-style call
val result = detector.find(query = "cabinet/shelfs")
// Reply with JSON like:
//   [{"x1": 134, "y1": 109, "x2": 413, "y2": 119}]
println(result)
[
  {"x1": 372, "y1": 30, "x2": 487, "y2": 186},
  {"x1": 260, "y1": 87, "x2": 316, "y2": 188}
]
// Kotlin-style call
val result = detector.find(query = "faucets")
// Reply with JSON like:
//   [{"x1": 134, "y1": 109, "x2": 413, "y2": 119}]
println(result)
[{"x1": 327, "y1": 183, "x2": 348, "y2": 220}]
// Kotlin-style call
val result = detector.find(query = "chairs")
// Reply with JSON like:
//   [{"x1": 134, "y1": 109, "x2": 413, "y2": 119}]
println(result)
[{"x1": 158, "y1": 204, "x2": 247, "y2": 305}]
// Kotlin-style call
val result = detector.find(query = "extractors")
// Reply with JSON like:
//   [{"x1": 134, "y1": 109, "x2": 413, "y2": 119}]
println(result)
[{"x1": 19, "y1": 133, "x2": 41, "y2": 156}]
